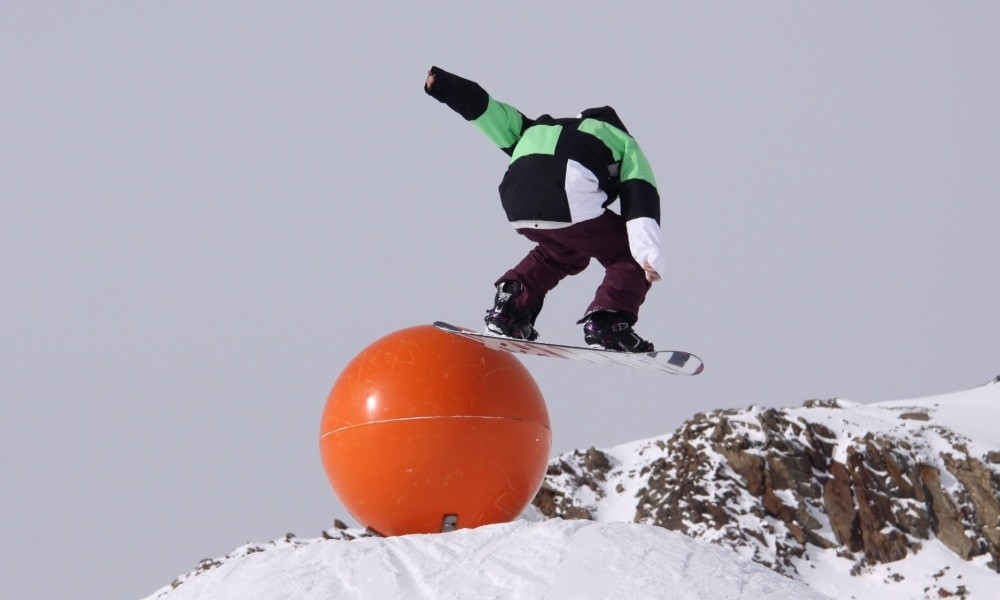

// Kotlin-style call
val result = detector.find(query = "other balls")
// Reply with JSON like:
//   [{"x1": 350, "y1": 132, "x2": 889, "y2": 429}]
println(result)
[{"x1": 322, "y1": 326, "x2": 551, "y2": 538}]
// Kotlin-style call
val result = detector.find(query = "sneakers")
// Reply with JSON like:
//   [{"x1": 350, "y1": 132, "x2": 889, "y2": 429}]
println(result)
[
  {"x1": 583, "y1": 309, "x2": 653, "y2": 353},
  {"x1": 486, "y1": 281, "x2": 539, "y2": 342}
]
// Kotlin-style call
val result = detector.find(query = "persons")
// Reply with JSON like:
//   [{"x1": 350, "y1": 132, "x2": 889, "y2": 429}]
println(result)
[{"x1": 424, "y1": 65, "x2": 665, "y2": 353}]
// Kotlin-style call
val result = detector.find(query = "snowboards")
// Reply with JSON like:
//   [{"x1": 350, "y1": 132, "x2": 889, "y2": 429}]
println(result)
[{"x1": 434, "y1": 319, "x2": 705, "y2": 377}]
